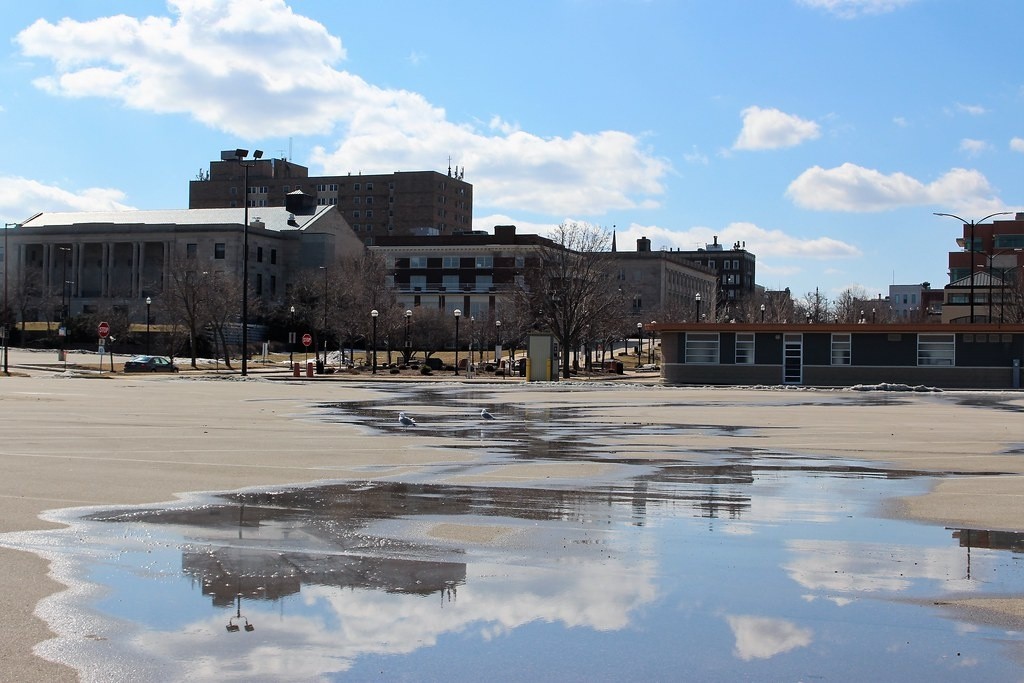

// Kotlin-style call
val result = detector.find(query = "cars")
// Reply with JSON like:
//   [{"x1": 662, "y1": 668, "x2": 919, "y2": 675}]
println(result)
[{"x1": 124, "y1": 356, "x2": 181, "y2": 373}]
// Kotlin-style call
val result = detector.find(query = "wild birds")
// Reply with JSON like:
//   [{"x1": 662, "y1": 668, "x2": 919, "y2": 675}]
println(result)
[
  {"x1": 481, "y1": 409, "x2": 495, "y2": 423},
  {"x1": 397, "y1": 412, "x2": 415, "y2": 428}
]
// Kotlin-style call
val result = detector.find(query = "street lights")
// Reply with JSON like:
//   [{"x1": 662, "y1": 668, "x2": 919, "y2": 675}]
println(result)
[
  {"x1": 695, "y1": 292, "x2": 701, "y2": 322},
  {"x1": 4, "y1": 222, "x2": 24, "y2": 373},
  {"x1": 370, "y1": 309, "x2": 379, "y2": 374},
  {"x1": 471, "y1": 316, "x2": 475, "y2": 366},
  {"x1": 66, "y1": 280, "x2": 74, "y2": 316},
  {"x1": 454, "y1": 309, "x2": 463, "y2": 377},
  {"x1": 496, "y1": 321, "x2": 502, "y2": 347},
  {"x1": 931, "y1": 210, "x2": 1014, "y2": 324},
  {"x1": 60, "y1": 247, "x2": 71, "y2": 327},
  {"x1": 289, "y1": 306, "x2": 295, "y2": 370},
  {"x1": 406, "y1": 310, "x2": 412, "y2": 366},
  {"x1": 637, "y1": 322, "x2": 643, "y2": 367},
  {"x1": 146, "y1": 297, "x2": 152, "y2": 340},
  {"x1": 234, "y1": 148, "x2": 264, "y2": 376},
  {"x1": 651, "y1": 321, "x2": 657, "y2": 364},
  {"x1": 758, "y1": 302, "x2": 930, "y2": 324},
  {"x1": 963, "y1": 246, "x2": 1024, "y2": 325}
]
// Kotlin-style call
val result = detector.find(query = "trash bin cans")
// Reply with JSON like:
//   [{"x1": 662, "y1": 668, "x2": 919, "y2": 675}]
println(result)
[{"x1": 520, "y1": 358, "x2": 526, "y2": 377}]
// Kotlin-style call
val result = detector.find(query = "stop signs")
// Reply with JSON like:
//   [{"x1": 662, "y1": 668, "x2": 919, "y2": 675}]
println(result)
[
  {"x1": 98, "y1": 322, "x2": 110, "y2": 338},
  {"x1": 302, "y1": 334, "x2": 312, "y2": 346}
]
